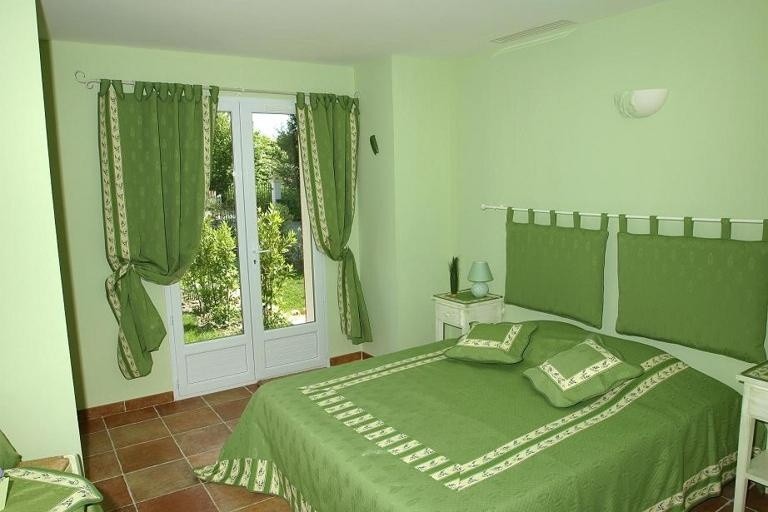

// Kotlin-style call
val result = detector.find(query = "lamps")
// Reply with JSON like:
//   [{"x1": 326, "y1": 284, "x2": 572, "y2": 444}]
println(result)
[{"x1": 467, "y1": 261, "x2": 495, "y2": 298}]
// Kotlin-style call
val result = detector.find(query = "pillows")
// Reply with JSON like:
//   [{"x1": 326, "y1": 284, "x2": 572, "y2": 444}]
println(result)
[
  {"x1": 444, "y1": 321, "x2": 537, "y2": 364},
  {"x1": 523, "y1": 334, "x2": 645, "y2": 408}
]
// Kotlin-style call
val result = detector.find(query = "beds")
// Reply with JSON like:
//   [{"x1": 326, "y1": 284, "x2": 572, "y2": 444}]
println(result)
[{"x1": 246, "y1": 316, "x2": 768, "y2": 511}]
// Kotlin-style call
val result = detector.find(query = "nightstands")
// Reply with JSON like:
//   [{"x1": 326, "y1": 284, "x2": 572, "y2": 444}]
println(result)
[
  {"x1": 432, "y1": 289, "x2": 504, "y2": 341},
  {"x1": 734, "y1": 360, "x2": 768, "y2": 512}
]
